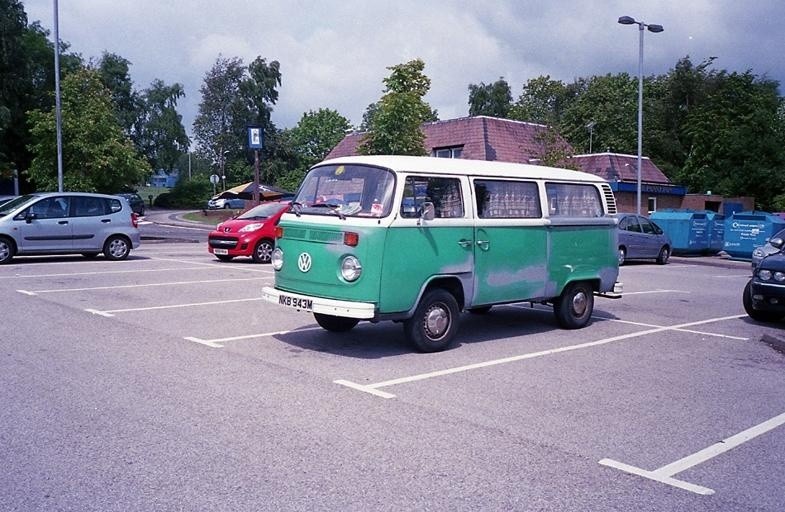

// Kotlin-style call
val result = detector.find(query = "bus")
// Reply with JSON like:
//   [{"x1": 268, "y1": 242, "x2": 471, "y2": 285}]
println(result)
[{"x1": 260, "y1": 154, "x2": 621, "y2": 353}]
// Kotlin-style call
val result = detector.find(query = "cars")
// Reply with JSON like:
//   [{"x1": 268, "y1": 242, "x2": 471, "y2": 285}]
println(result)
[
  {"x1": 208, "y1": 195, "x2": 244, "y2": 209},
  {"x1": 0, "y1": 190, "x2": 142, "y2": 263},
  {"x1": 207, "y1": 201, "x2": 293, "y2": 263},
  {"x1": 620, "y1": 210, "x2": 674, "y2": 265},
  {"x1": 741, "y1": 226, "x2": 785, "y2": 329}
]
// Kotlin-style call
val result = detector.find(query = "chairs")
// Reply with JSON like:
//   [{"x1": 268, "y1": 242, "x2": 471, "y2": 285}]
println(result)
[{"x1": 426, "y1": 178, "x2": 463, "y2": 218}]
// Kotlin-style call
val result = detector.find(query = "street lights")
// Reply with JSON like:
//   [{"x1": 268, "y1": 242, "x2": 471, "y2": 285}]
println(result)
[{"x1": 617, "y1": 15, "x2": 665, "y2": 216}]
